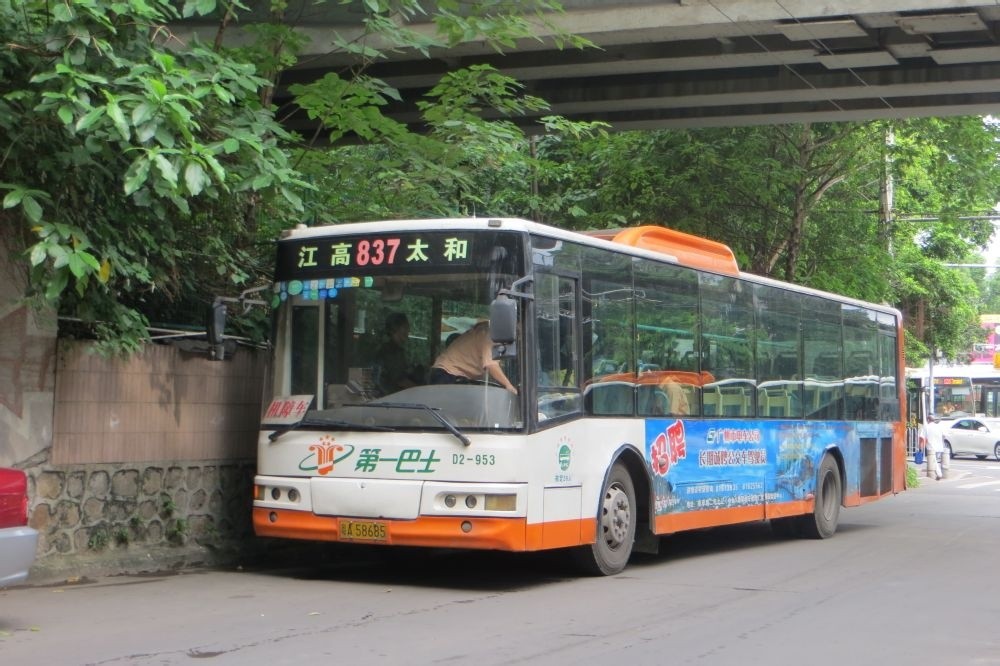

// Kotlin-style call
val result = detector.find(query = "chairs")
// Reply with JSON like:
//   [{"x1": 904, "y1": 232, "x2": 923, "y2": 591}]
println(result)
[{"x1": 592, "y1": 349, "x2": 874, "y2": 420}]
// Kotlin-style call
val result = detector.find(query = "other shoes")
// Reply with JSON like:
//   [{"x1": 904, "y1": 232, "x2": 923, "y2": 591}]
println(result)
[{"x1": 936, "y1": 475, "x2": 941, "y2": 480}]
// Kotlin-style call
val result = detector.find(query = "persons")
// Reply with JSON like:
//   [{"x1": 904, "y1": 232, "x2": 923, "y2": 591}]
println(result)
[
  {"x1": 431, "y1": 321, "x2": 518, "y2": 394},
  {"x1": 919, "y1": 415, "x2": 942, "y2": 480},
  {"x1": 943, "y1": 400, "x2": 955, "y2": 415},
  {"x1": 650, "y1": 355, "x2": 690, "y2": 415},
  {"x1": 370, "y1": 313, "x2": 413, "y2": 395}
]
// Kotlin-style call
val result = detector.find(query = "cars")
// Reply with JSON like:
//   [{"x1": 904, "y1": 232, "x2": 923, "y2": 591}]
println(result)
[
  {"x1": 0, "y1": 468, "x2": 38, "y2": 587},
  {"x1": 937, "y1": 416, "x2": 1000, "y2": 460}
]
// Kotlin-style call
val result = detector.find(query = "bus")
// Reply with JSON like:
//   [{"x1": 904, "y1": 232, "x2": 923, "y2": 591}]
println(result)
[
  {"x1": 205, "y1": 215, "x2": 906, "y2": 577},
  {"x1": 905, "y1": 364, "x2": 999, "y2": 423}
]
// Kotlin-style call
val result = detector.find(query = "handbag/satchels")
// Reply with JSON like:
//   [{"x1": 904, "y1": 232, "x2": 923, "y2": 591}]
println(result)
[{"x1": 915, "y1": 450, "x2": 923, "y2": 464}]
